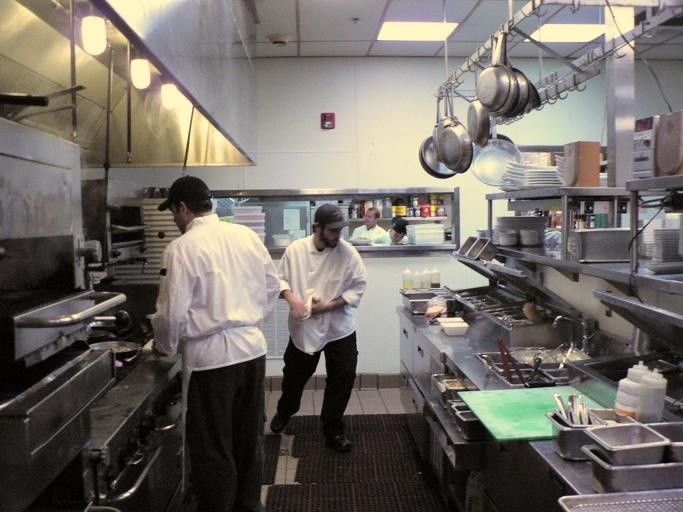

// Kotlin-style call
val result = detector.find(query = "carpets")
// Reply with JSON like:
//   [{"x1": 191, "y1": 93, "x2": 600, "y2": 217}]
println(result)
[{"x1": 256, "y1": 412, "x2": 458, "y2": 512}]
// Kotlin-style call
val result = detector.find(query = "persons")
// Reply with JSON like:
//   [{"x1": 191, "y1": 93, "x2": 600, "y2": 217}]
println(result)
[
  {"x1": 270, "y1": 204, "x2": 367, "y2": 451},
  {"x1": 348, "y1": 207, "x2": 386, "y2": 243},
  {"x1": 382, "y1": 218, "x2": 410, "y2": 243},
  {"x1": 151, "y1": 174, "x2": 280, "y2": 512}
]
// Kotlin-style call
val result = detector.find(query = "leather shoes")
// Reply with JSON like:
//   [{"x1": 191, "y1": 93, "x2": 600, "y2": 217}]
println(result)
[
  {"x1": 270, "y1": 413, "x2": 290, "y2": 433},
  {"x1": 327, "y1": 435, "x2": 351, "y2": 450}
]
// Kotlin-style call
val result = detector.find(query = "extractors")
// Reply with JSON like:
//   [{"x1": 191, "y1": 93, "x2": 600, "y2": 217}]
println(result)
[{"x1": 0, "y1": 1, "x2": 255, "y2": 167}]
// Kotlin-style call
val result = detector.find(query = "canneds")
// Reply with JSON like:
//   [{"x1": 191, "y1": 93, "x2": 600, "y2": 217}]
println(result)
[{"x1": 408, "y1": 198, "x2": 421, "y2": 216}]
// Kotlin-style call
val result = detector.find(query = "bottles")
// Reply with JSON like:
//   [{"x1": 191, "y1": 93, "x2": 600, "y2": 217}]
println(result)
[
  {"x1": 423, "y1": 267, "x2": 432, "y2": 289},
  {"x1": 550, "y1": 210, "x2": 587, "y2": 230},
  {"x1": 402, "y1": 267, "x2": 413, "y2": 290},
  {"x1": 413, "y1": 270, "x2": 422, "y2": 288},
  {"x1": 430, "y1": 267, "x2": 440, "y2": 287},
  {"x1": 614, "y1": 359, "x2": 668, "y2": 421},
  {"x1": 374, "y1": 194, "x2": 408, "y2": 218}
]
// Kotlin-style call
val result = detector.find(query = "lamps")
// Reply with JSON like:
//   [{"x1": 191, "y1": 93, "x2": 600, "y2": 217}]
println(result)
[
  {"x1": 157, "y1": 80, "x2": 181, "y2": 108},
  {"x1": 79, "y1": 2, "x2": 110, "y2": 60},
  {"x1": 126, "y1": 51, "x2": 155, "y2": 89}
]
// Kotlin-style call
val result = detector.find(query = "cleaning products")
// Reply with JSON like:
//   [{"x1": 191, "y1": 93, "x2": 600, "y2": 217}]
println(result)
[
  {"x1": 402, "y1": 265, "x2": 441, "y2": 289},
  {"x1": 612, "y1": 359, "x2": 667, "y2": 424}
]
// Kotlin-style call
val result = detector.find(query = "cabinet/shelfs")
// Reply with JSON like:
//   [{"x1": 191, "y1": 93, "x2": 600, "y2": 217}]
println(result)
[
  {"x1": 522, "y1": 436, "x2": 610, "y2": 511},
  {"x1": 139, "y1": 364, "x2": 187, "y2": 511},
  {"x1": 80, "y1": 406, "x2": 160, "y2": 511},
  {"x1": 392, "y1": 304, "x2": 447, "y2": 474}
]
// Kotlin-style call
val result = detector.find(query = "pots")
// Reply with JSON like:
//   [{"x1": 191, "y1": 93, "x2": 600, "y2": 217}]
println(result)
[
  {"x1": 417, "y1": 29, "x2": 539, "y2": 188},
  {"x1": 89, "y1": 341, "x2": 145, "y2": 359}
]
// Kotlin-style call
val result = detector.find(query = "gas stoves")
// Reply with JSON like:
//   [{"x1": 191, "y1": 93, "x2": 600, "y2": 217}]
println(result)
[{"x1": 91, "y1": 328, "x2": 186, "y2": 473}]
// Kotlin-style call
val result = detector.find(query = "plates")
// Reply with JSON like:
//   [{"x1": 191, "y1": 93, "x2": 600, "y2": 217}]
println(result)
[
  {"x1": 348, "y1": 239, "x2": 373, "y2": 245},
  {"x1": 476, "y1": 226, "x2": 539, "y2": 246},
  {"x1": 636, "y1": 217, "x2": 680, "y2": 263},
  {"x1": 498, "y1": 157, "x2": 565, "y2": 190}
]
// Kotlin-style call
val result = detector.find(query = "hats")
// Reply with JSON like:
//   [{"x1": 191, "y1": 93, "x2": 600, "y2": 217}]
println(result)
[
  {"x1": 158, "y1": 175, "x2": 213, "y2": 211},
  {"x1": 315, "y1": 204, "x2": 350, "y2": 230}
]
